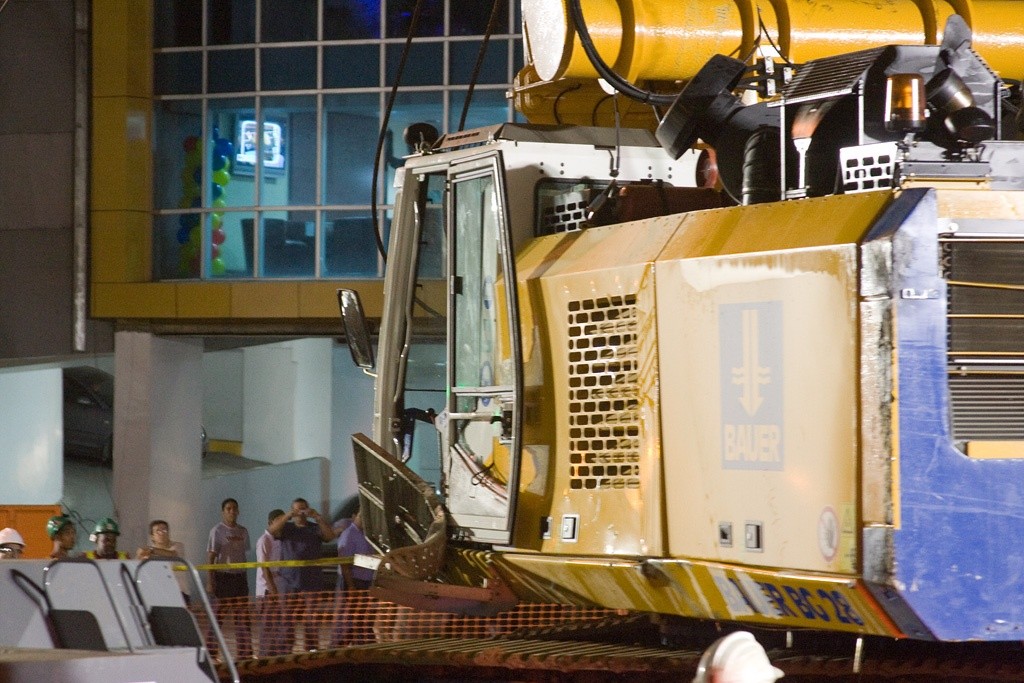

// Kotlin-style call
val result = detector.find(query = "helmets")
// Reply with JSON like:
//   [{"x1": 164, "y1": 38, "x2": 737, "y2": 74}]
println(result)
[
  {"x1": 94, "y1": 517, "x2": 120, "y2": 536},
  {"x1": 688, "y1": 630, "x2": 785, "y2": 683},
  {"x1": 0, "y1": 528, "x2": 25, "y2": 547},
  {"x1": 47, "y1": 516, "x2": 74, "y2": 537}
]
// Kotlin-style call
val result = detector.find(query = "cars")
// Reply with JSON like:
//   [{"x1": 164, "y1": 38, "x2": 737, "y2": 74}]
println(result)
[{"x1": 63, "y1": 365, "x2": 207, "y2": 465}]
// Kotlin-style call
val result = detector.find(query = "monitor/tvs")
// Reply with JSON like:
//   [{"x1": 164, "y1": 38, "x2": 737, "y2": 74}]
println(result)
[{"x1": 230, "y1": 110, "x2": 290, "y2": 178}]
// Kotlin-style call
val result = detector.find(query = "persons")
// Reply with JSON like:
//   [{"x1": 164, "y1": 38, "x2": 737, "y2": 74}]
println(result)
[
  {"x1": 205, "y1": 498, "x2": 254, "y2": 664},
  {"x1": 256, "y1": 498, "x2": 378, "y2": 655},
  {"x1": 137, "y1": 520, "x2": 184, "y2": 559},
  {"x1": 0, "y1": 527, "x2": 27, "y2": 558},
  {"x1": 47, "y1": 517, "x2": 75, "y2": 558},
  {"x1": 78, "y1": 517, "x2": 132, "y2": 559}
]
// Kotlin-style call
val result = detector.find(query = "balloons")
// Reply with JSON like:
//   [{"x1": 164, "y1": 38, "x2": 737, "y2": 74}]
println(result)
[{"x1": 178, "y1": 125, "x2": 233, "y2": 277}]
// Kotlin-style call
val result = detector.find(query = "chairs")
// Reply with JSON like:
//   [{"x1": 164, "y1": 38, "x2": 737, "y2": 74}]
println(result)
[{"x1": 241, "y1": 218, "x2": 315, "y2": 273}]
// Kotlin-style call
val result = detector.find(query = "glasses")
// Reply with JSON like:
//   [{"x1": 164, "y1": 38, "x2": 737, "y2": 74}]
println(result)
[
  {"x1": 153, "y1": 530, "x2": 168, "y2": 535},
  {"x1": 0, "y1": 547, "x2": 23, "y2": 556}
]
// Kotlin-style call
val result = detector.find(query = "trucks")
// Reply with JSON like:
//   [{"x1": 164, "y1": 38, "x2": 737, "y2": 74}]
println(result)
[{"x1": 336, "y1": 0, "x2": 1024, "y2": 641}]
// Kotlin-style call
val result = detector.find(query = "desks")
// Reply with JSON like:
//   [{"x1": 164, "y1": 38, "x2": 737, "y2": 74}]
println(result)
[{"x1": 328, "y1": 216, "x2": 392, "y2": 276}]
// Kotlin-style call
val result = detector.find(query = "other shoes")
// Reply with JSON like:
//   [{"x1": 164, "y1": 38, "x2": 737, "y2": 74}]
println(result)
[
  {"x1": 210, "y1": 658, "x2": 222, "y2": 665},
  {"x1": 235, "y1": 654, "x2": 259, "y2": 659},
  {"x1": 306, "y1": 646, "x2": 317, "y2": 652}
]
